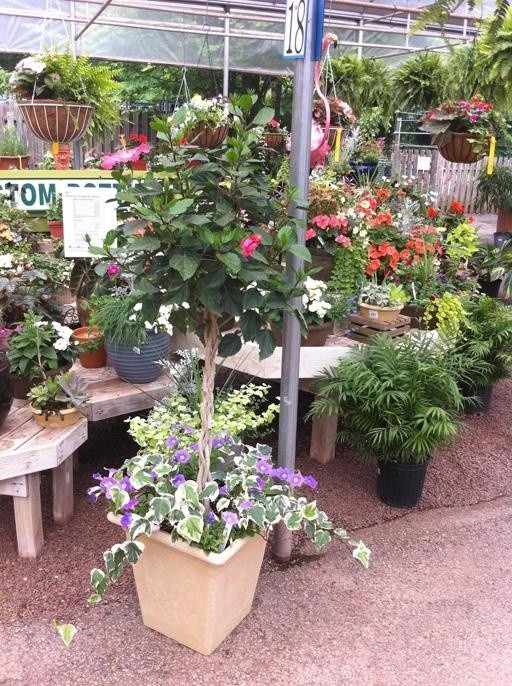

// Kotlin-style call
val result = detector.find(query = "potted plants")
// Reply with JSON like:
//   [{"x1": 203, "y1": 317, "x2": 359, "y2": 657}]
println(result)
[
  {"x1": 453, "y1": 301, "x2": 511, "y2": 413},
  {"x1": 315, "y1": 48, "x2": 447, "y2": 184},
  {"x1": 472, "y1": 163, "x2": 512, "y2": 235},
  {"x1": 358, "y1": 283, "x2": 404, "y2": 325},
  {"x1": 306, "y1": 329, "x2": 495, "y2": 508},
  {"x1": 1, "y1": 128, "x2": 32, "y2": 173},
  {"x1": 45, "y1": 191, "x2": 68, "y2": 242},
  {"x1": 8, "y1": 40, "x2": 125, "y2": 143},
  {"x1": 13, "y1": 311, "x2": 94, "y2": 431}
]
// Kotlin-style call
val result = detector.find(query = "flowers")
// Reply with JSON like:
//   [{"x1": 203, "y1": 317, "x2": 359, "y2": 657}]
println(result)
[
  {"x1": 191, "y1": 94, "x2": 223, "y2": 114},
  {"x1": 356, "y1": 175, "x2": 442, "y2": 276},
  {"x1": 268, "y1": 118, "x2": 280, "y2": 130},
  {"x1": 426, "y1": 96, "x2": 506, "y2": 123},
  {"x1": 86, "y1": 142, "x2": 370, "y2": 531},
  {"x1": 449, "y1": 200, "x2": 475, "y2": 225}
]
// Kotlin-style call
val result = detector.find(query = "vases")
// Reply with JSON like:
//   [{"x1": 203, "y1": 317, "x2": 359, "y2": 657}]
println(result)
[
  {"x1": 261, "y1": 133, "x2": 289, "y2": 149},
  {"x1": 436, "y1": 130, "x2": 497, "y2": 163},
  {"x1": 130, "y1": 522, "x2": 269, "y2": 655},
  {"x1": 103, "y1": 329, "x2": 172, "y2": 385},
  {"x1": 73, "y1": 325, "x2": 108, "y2": 369},
  {"x1": 183, "y1": 120, "x2": 229, "y2": 150}
]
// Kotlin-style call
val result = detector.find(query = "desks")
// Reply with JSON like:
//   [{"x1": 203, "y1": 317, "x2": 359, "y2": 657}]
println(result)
[{"x1": 0, "y1": 398, "x2": 87, "y2": 559}]
